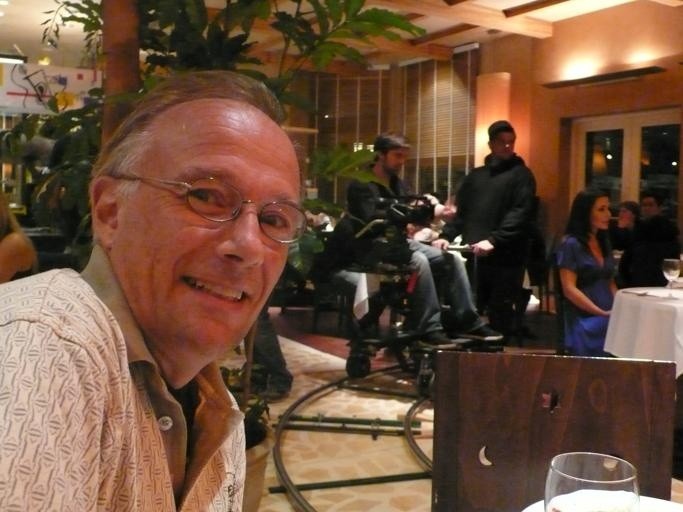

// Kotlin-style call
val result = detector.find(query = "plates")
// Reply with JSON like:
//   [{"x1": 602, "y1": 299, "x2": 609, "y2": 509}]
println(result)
[{"x1": 446, "y1": 245, "x2": 472, "y2": 252}]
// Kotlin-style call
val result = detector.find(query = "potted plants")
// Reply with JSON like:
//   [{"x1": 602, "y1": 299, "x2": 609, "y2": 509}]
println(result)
[{"x1": 0, "y1": 0, "x2": 428, "y2": 511}]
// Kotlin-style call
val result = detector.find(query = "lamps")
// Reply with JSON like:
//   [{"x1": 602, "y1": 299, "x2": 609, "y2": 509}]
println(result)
[{"x1": 0, "y1": 53, "x2": 28, "y2": 65}]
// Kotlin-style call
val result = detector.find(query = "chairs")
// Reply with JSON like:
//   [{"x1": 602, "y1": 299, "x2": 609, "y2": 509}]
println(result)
[
  {"x1": 309, "y1": 231, "x2": 356, "y2": 334},
  {"x1": 553, "y1": 269, "x2": 614, "y2": 355},
  {"x1": 432, "y1": 350, "x2": 677, "y2": 512}
]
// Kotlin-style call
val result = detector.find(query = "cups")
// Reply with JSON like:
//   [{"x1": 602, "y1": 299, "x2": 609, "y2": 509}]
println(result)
[{"x1": 542, "y1": 448, "x2": 638, "y2": 512}]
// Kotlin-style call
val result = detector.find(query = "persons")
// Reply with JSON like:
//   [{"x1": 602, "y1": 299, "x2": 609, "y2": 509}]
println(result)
[
  {"x1": 0, "y1": 190, "x2": 37, "y2": 281},
  {"x1": 0, "y1": 71, "x2": 308, "y2": 512},
  {"x1": 0, "y1": 70, "x2": 683, "y2": 394},
  {"x1": 343, "y1": 130, "x2": 505, "y2": 350},
  {"x1": 435, "y1": 119, "x2": 535, "y2": 342},
  {"x1": 554, "y1": 188, "x2": 618, "y2": 356}
]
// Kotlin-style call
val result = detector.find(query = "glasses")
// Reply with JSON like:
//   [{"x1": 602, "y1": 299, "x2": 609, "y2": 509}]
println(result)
[{"x1": 110, "y1": 170, "x2": 309, "y2": 244}]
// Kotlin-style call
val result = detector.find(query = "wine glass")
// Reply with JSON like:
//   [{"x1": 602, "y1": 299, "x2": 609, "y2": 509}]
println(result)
[{"x1": 660, "y1": 259, "x2": 679, "y2": 301}]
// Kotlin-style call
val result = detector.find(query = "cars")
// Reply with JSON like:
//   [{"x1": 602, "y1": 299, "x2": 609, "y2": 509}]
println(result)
[
  {"x1": 648, "y1": 173, "x2": 676, "y2": 190},
  {"x1": 595, "y1": 170, "x2": 649, "y2": 193}
]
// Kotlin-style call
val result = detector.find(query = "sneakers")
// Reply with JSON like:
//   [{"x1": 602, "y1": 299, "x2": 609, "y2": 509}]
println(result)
[
  {"x1": 260, "y1": 391, "x2": 284, "y2": 402},
  {"x1": 418, "y1": 333, "x2": 457, "y2": 349},
  {"x1": 457, "y1": 326, "x2": 504, "y2": 341}
]
